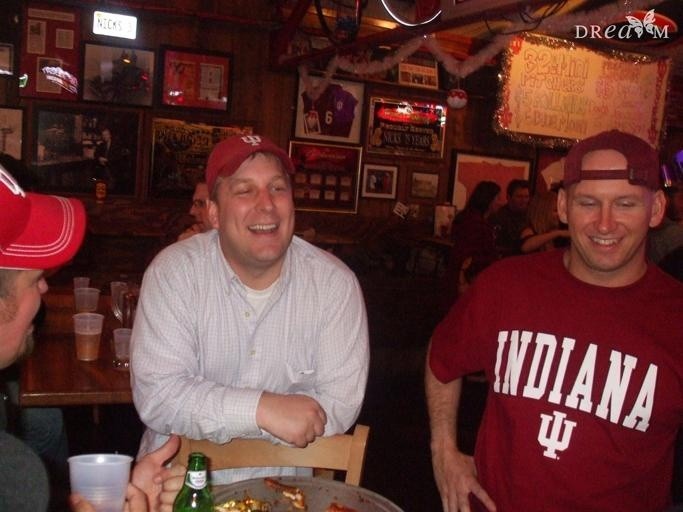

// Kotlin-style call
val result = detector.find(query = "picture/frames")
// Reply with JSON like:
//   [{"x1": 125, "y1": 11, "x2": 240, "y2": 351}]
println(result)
[
  {"x1": 80, "y1": 42, "x2": 158, "y2": 109},
  {"x1": 157, "y1": 44, "x2": 234, "y2": 115},
  {"x1": 292, "y1": 70, "x2": 363, "y2": 144},
  {"x1": 287, "y1": 141, "x2": 361, "y2": 218},
  {"x1": 363, "y1": 94, "x2": 533, "y2": 238},
  {"x1": 0, "y1": 103, "x2": 26, "y2": 164},
  {"x1": 23, "y1": 101, "x2": 146, "y2": 203},
  {"x1": 0, "y1": 41, "x2": 14, "y2": 79}
]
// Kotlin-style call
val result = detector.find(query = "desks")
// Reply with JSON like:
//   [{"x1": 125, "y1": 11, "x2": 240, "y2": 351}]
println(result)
[{"x1": 18, "y1": 267, "x2": 147, "y2": 424}]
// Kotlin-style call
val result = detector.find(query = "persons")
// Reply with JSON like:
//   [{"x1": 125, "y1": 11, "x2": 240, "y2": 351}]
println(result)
[
  {"x1": 649, "y1": 188, "x2": 682, "y2": 276},
  {"x1": 448, "y1": 178, "x2": 567, "y2": 302},
  {"x1": 300, "y1": 69, "x2": 361, "y2": 139},
  {"x1": 93, "y1": 127, "x2": 117, "y2": 180},
  {"x1": 0, "y1": 163, "x2": 187, "y2": 512},
  {"x1": 427, "y1": 129, "x2": 682, "y2": 511},
  {"x1": 129, "y1": 131, "x2": 371, "y2": 485},
  {"x1": 177, "y1": 175, "x2": 212, "y2": 241}
]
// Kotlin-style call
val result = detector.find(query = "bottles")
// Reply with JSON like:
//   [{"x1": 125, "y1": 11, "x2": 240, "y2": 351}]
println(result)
[
  {"x1": 171, "y1": 450, "x2": 216, "y2": 512},
  {"x1": 109, "y1": 280, "x2": 139, "y2": 327}
]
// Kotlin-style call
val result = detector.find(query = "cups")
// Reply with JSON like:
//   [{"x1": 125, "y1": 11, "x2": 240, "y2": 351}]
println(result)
[
  {"x1": 65, "y1": 452, "x2": 135, "y2": 512},
  {"x1": 73, "y1": 277, "x2": 104, "y2": 363},
  {"x1": 94, "y1": 180, "x2": 106, "y2": 205},
  {"x1": 110, "y1": 328, "x2": 132, "y2": 372}
]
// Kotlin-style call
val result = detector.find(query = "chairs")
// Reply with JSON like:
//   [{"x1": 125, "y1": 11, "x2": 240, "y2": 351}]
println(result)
[{"x1": 165, "y1": 423, "x2": 368, "y2": 487}]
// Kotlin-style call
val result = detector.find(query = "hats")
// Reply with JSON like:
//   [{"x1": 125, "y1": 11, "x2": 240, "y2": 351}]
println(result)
[
  {"x1": 564, "y1": 129, "x2": 659, "y2": 187},
  {"x1": 0, "y1": 164, "x2": 87, "y2": 270},
  {"x1": 205, "y1": 134, "x2": 295, "y2": 194}
]
